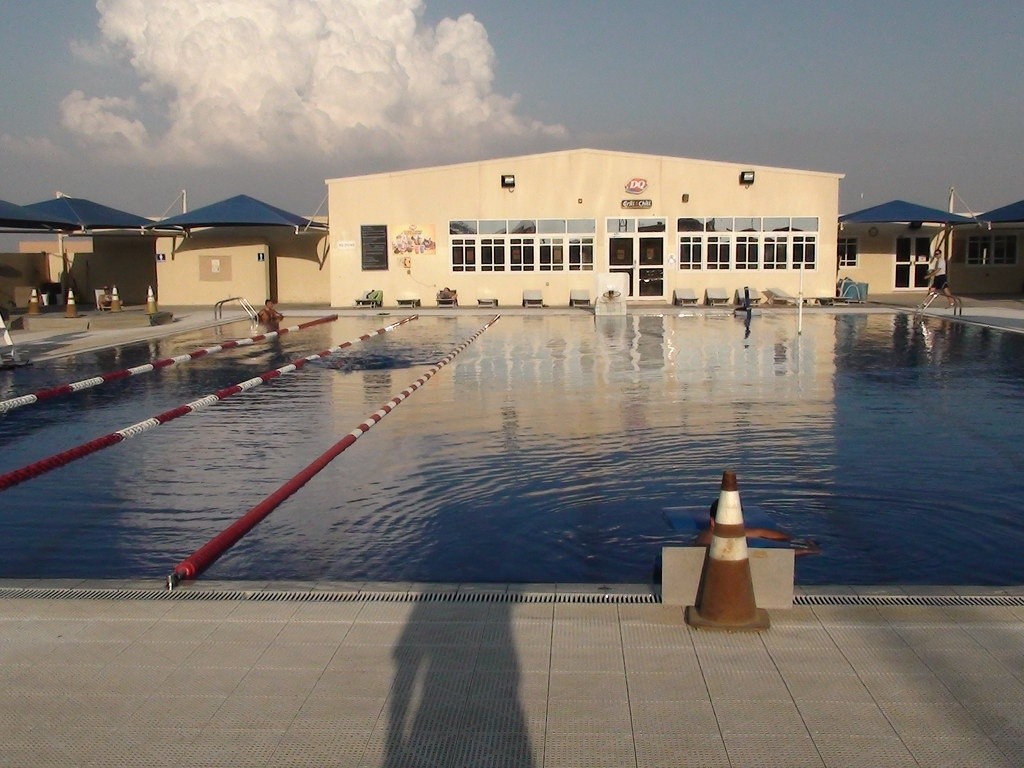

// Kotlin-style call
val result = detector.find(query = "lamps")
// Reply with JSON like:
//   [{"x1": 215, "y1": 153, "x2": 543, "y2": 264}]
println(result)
[
  {"x1": 741, "y1": 171, "x2": 755, "y2": 185},
  {"x1": 909, "y1": 220, "x2": 923, "y2": 230},
  {"x1": 501, "y1": 175, "x2": 515, "y2": 189}
]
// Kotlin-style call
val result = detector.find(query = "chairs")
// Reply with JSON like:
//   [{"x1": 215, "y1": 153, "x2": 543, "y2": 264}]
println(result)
[
  {"x1": 396, "y1": 299, "x2": 421, "y2": 308},
  {"x1": 436, "y1": 289, "x2": 458, "y2": 308},
  {"x1": 736, "y1": 288, "x2": 762, "y2": 307},
  {"x1": 353, "y1": 290, "x2": 383, "y2": 308},
  {"x1": 477, "y1": 298, "x2": 498, "y2": 309},
  {"x1": 94, "y1": 289, "x2": 126, "y2": 311},
  {"x1": 673, "y1": 289, "x2": 700, "y2": 307},
  {"x1": 706, "y1": 288, "x2": 730, "y2": 308},
  {"x1": 765, "y1": 288, "x2": 852, "y2": 307},
  {"x1": 523, "y1": 290, "x2": 544, "y2": 308},
  {"x1": 570, "y1": 289, "x2": 592, "y2": 308}
]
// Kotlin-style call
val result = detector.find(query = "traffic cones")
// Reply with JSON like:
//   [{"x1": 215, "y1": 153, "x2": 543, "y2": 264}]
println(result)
[
  {"x1": 144, "y1": 286, "x2": 158, "y2": 315},
  {"x1": 63, "y1": 288, "x2": 80, "y2": 318},
  {"x1": 109, "y1": 284, "x2": 123, "y2": 312},
  {"x1": 28, "y1": 289, "x2": 42, "y2": 316},
  {"x1": 684, "y1": 469, "x2": 772, "y2": 632}
]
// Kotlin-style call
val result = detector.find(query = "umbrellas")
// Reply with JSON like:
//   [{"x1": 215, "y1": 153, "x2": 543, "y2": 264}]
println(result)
[
  {"x1": 0, "y1": 199, "x2": 71, "y2": 229},
  {"x1": 971, "y1": 200, "x2": 1024, "y2": 223},
  {"x1": 144, "y1": 193, "x2": 328, "y2": 234},
  {"x1": 839, "y1": 199, "x2": 977, "y2": 226},
  {"x1": 22, "y1": 196, "x2": 182, "y2": 236}
]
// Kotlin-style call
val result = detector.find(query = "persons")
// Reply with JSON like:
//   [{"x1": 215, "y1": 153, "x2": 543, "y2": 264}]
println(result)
[
  {"x1": 916, "y1": 249, "x2": 956, "y2": 306},
  {"x1": 98, "y1": 285, "x2": 124, "y2": 307},
  {"x1": 437, "y1": 287, "x2": 458, "y2": 299},
  {"x1": 696, "y1": 498, "x2": 823, "y2": 555},
  {"x1": 255, "y1": 299, "x2": 283, "y2": 319}
]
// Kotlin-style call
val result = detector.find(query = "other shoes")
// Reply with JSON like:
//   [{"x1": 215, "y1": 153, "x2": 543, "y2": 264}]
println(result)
[
  {"x1": 949, "y1": 300, "x2": 958, "y2": 306},
  {"x1": 916, "y1": 302, "x2": 928, "y2": 308}
]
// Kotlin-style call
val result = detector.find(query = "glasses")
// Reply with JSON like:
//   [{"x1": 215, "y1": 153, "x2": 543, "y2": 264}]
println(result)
[{"x1": 934, "y1": 253, "x2": 940, "y2": 255}]
